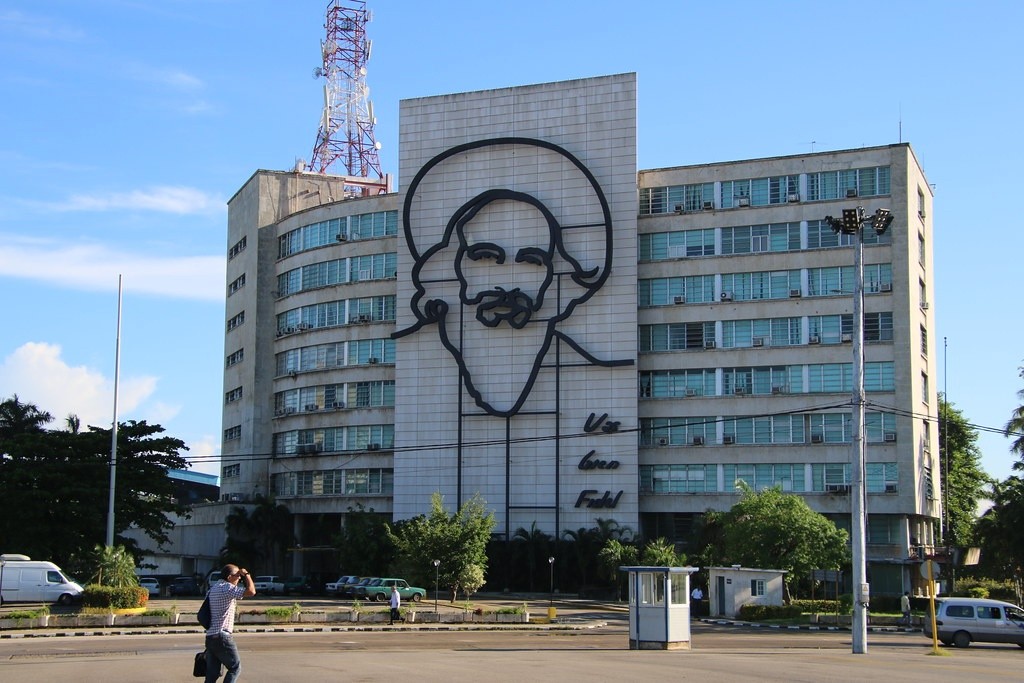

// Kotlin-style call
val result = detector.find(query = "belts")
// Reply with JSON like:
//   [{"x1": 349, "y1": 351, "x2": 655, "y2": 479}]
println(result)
[{"x1": 206, "y1": 635, "x2": 222, "y2": 641}]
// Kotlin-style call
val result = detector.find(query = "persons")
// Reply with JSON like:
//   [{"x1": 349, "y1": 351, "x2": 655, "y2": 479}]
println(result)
[
  {"x1": 387, "y1": 586, "x2": 405, "y2": 625},
  {"x1": 897, "y1": 591, "x2": 913, "y2": 628},
  {"x1": 691, "y1": 585, "x2": 704, "y2": 618},
  {"x1": 204, "y1": 565, "x2": 256, "y2": 683}
]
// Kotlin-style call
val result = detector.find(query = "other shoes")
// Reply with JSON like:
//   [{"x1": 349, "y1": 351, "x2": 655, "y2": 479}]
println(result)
[
  {"x1": 401, "y1": 618, "x2": 405, "y2": 624},
  {"x1": 387, "y1": 622, "x2": 394, "y2": 625}
]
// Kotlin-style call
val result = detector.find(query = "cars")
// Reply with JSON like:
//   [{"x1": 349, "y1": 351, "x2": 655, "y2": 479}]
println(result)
[
  {"x1": 337, "y1": 577, "x2": 368, "y2": 600},
  {"x1": 138, "y1": 578, "x2": 161, "y2": 598},
  {"x1": 325, "y1": 576, "x2": 357, "y2": 597},
  {"x1": 253, "y1": 576, "x2": 285, "y2": 596},
  {"x1": 283, "y1": 576, "x2": 324, "y2": 597},
  {"x1": 206, "y1": 572, "x2": 222, "y2": 591},
  {"x1": 352, "y1": 578, "x2": 382, "y2": 600},
  {"x1": 923, "y1": 597, "x2": 1024, "y2": 649},
  {"x1": 169, "y1": 576, "x2": 204, "y2": 596},
  {"x1": 360, "y1": 578, "x2": 426, "y2": 601}
]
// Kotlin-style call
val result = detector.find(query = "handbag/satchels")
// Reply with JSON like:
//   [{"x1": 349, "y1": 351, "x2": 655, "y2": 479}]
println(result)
[
  {"x1": 196, "y1": 588, "x2": 212, "y2": 630},
  {"x1": 393, "y1": 611, "x2": 400, "y2": 620},
  {"x1": 192, "y1": 647, "x2": 225, "y2": 678}
]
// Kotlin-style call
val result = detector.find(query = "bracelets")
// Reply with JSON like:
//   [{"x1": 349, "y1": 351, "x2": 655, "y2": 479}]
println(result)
[{"x1": 245, "y1": 572, "x2": 250, "y2": 576}]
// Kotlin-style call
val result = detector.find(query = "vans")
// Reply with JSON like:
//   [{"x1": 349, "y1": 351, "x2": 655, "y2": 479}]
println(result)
[{"x1": 0, "y1": 554, "x2": 84, "y2": 605}]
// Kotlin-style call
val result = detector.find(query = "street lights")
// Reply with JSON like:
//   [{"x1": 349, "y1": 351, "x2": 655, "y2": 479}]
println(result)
[
  {"x1": 434, "y1": 560, "x2": 440, "y2": 612},
  {"x1": 824, "y1": 206, "x2": 895, "y2": 654},
  {"x1": 549, "y1": 557, "x2": 554, "y2": 607}
]
